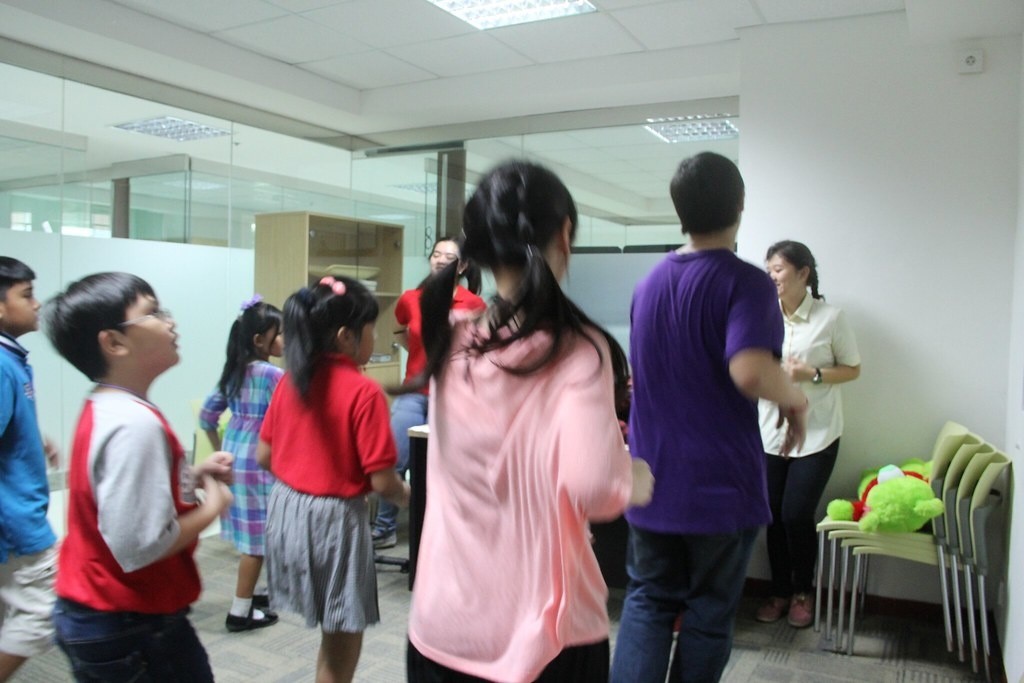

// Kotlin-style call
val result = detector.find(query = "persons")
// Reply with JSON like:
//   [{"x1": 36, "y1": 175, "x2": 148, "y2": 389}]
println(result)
[
  {"x1": 252, "y1": 274, "x2": 411, "y2": 683},
  {"x1": 404, "y1": 158, "x2": 655, "y2": 683},
  {"x1": 0, "y1": 254, "x2": 65, "y2": 683},
  {"x1": 48, "y1": 273, "x2": 238, "y2": 683},
  {"x1": 756, "y1": 240, "x2": 863, "y2": 627},
  {"x1": 199, "y1": 293, "x2": 285, "y2": 630},
  {"x1": 370, "y1": 236, "x2": 488, "y2": 550},
  {"x1": 610, "y1": 149, "x2": 810, "y2": 683}
]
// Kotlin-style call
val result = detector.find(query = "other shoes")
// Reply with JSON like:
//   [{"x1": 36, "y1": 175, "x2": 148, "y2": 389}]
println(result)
[{"x1": 225, "y1": 593, "x2": 279, "y2": 631}]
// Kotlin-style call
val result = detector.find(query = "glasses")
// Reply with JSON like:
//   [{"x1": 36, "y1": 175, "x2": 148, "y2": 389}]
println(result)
[{"x1": 118, "y1": 309, "x2": 178, "y2": 327}]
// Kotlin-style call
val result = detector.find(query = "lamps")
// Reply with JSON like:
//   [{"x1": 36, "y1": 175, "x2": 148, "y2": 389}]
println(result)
[
  {"x1": 643, "y1": 120, "x2": 740, "y2": 143},
  {"x1": 112, "y1": 116, "x2": 237, "y2": 144}
]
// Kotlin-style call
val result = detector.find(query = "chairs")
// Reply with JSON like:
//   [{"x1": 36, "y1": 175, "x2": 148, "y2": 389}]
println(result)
[{"x1": 813, "y1": 421, "x2": 1013, "y2": 683}]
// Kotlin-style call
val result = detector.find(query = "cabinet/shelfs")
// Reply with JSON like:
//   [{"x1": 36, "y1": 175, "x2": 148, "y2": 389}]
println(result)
[{"x1": 252, "y1": 211, "x2": 405, "y2": 543}]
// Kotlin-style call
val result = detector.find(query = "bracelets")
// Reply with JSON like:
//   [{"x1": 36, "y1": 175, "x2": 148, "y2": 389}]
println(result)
[{"x1": 813, "y1": 367, "x2": 822, "y2": 385}]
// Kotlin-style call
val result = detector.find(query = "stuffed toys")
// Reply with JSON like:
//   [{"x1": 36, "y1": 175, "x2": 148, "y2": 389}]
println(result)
[{"x1": 826, "y1": 456, "x2": 944, "y2": 535}]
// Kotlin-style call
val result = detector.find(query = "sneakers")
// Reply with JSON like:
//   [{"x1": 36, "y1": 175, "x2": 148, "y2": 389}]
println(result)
[
  {"x1": 371, "y1": 528, "x2": 398, "y2": 548},
  {"x1": 755, "y1": 596, "x2": 785, "y2": 622},
  {"x1": 787, "y1": 591, "x2": 815, "y2": 627}
]
